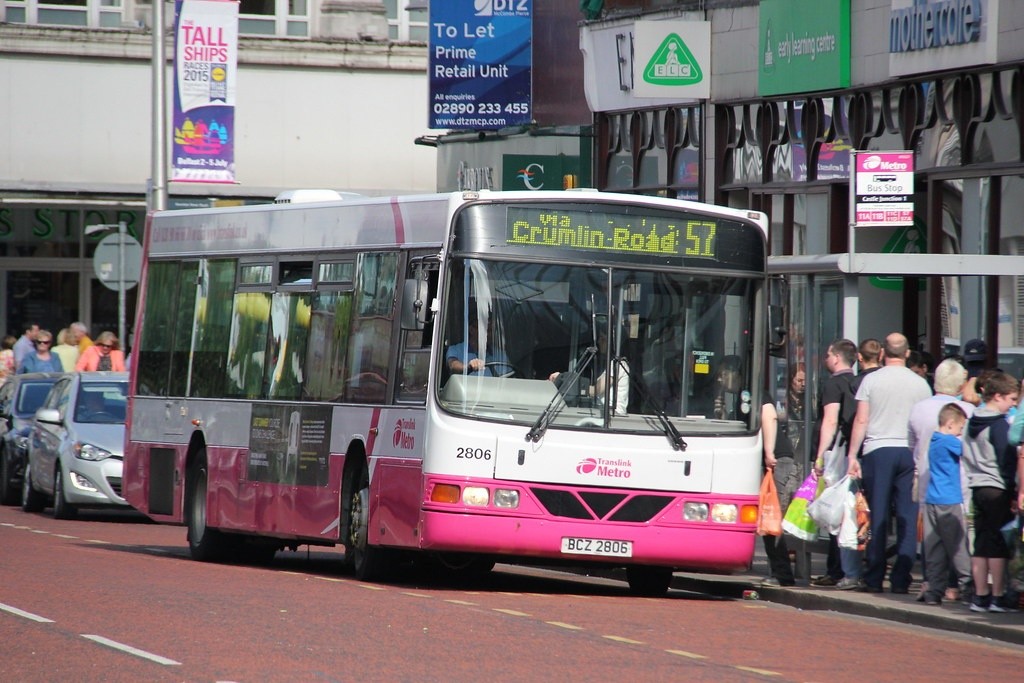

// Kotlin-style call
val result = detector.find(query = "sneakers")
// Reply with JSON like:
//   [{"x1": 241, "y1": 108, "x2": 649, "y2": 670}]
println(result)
[
  {"x1": 969, "y1": 594, "x2": 990, "y2": 612},
  {"x1": 989, "y1": 595, "x2": 1022, "y2": 614}
]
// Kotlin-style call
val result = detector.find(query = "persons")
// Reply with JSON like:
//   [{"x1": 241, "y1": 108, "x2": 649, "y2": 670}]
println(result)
[
  {"x1": 812, "y1": 339, "x2": 858, "y2": 585},
  {"x1": 847, "y1": 333, "x2": 932, "y2": 593},
  {"x1": 446, "y1": 318, "x2": 512, "y2": 377},
  {"x1": 836, "y1": 338, "x2": 884, "y2": 589},
  {"x1": 51, "y1": 322, "x2": 93, "y2": 372},
  {"x1": 789, "y1": 363, "x2": 805, "y2": 451},
  {"x1": 0, "y1": 322, "x2": 39, "y2": 386},
  {"x1": 16, "y1": 330, "x2": 64, "y2": 374},
  {"x1": 905, "y1": 339, "x2": 1024, "y2": 614},
  {"x1": 76, "y1": 331, "x2": 124, "y2": 373},
  {"x1": 550, "y1": 322, "x2": 630, "y2": 415},
  {"x1": 714, "y1": 355, "x2": 795, "y2": 588}
]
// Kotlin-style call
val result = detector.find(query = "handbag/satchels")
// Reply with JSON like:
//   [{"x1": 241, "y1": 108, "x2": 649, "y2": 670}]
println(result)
[
  {"x1": 782, "y1": 465, "x2": 826, "y2": 543},
  {"x1": 757, "y1": 469, "x2": 785, "y2": 538},
  {"x1": 835, "y1": 487, "x2": 860, "y2": 550},
  {"x1": 854, "y1": 489, "x2": 874, "y2": 551},
  {"x1": 805, "y1": 471, "x2": 853, "y2": 537},
  {"x1": 823, "y1": 433, "x2": 853, "y2": 487}
]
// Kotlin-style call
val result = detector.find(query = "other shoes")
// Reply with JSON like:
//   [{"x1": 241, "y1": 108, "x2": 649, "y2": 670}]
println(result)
[
  {"x1": 813, "y1": 576, "x2": 838, "y2": 586},
  {"x1": 889, "y1": 584, "x2": 909, "y2": 594},
  {"x1": 835, "y1": 577, "x2": 859, "y2": 592},
  {"x1": 923, "y1": 593, "x2": 942, "y2": 606},
  {"x1": 855, "y1": 582, "x2": 885, "y2": 595},
  {"x1": 916, "y1": 581, "x2": 931, "y2": 602},
  {"x1": 759, "y1": 576, "x2": 795, "y2": 588},
  {"x1": 941, "y1": 587, "x2": 959, "y2": 603}
]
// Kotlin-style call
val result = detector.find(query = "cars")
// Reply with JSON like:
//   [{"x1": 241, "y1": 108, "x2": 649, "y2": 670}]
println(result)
[
  {"x1": 0, "y1": 374, "x2": 66, "y2": 507},
  {"x1": 19, "y1": 371, "x2": 130, "y2": 520}
]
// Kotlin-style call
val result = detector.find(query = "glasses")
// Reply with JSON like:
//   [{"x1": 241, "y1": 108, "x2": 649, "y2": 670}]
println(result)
[
  {"x1": 100, "y1": 343, "x2": 111, "y2": 348},
  {"x1": 37, "y1": 340, "x2": 49, "y2": 345}
]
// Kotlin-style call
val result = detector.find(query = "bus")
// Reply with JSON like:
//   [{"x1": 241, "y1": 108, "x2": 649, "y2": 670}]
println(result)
[{"x1": 122, "y1": 189, "x2": 788, "y2": 599}]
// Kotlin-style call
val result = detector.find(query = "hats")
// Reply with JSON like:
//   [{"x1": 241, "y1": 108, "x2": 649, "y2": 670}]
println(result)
[{"x1": 962, "y1": 338, "x2": 987, "y2": 362}]
